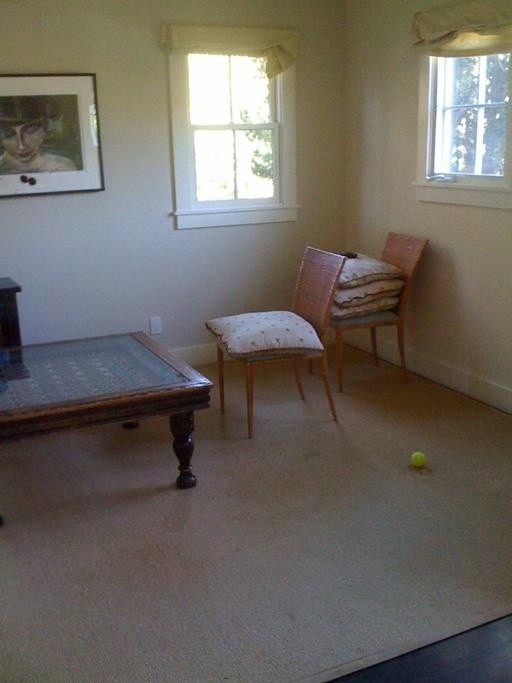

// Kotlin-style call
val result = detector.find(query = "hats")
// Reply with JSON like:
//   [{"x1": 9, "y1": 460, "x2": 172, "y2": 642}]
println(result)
[{"x1": 0, "y1": 94, "x2": 62, "y2": 125}]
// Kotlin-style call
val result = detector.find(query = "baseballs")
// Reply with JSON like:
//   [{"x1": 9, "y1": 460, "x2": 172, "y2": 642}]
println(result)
[{"x1": 411, "y1": 453, "x2": 427, "y2": 468}]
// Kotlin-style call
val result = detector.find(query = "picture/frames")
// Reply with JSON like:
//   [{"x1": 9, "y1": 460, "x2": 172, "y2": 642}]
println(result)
[{"x1": 0, "y1": 68, "x2": 106, "y2": 199}]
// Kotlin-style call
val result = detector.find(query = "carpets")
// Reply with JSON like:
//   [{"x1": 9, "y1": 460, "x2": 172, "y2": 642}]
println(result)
[{"x1": 1, "y1": 338, "x2": 512, "y2": 683}]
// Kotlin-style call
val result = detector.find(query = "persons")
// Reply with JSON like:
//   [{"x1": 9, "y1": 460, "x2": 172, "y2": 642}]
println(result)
[{"x1": 0, "y1": 96, "x2": 78, "y2": 175}]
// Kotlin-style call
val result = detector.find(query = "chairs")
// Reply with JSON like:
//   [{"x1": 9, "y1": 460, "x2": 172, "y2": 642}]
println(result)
[
  {"x1": 203, "y1": 243, "x2": 349, "y2": 438},
  {"x1": 308, "y1": 228, "x2": 430, "y2": 393}
]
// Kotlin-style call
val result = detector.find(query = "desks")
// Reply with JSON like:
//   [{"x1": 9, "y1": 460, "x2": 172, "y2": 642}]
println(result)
[
  {"x1": 0, "y1": 275, "x2": 30, "y2": 380},
  {"x1": 0, "y1": 327, "x2": 214, "y2": 529}
]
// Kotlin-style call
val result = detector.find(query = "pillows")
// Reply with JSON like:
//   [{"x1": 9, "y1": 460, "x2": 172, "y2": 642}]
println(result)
[
  {"x1": 332, "y1": 276, "x2": 406, "y2": 307},
  {"x1": 204, "y1": 307, "x2": 328, "y2": 362},
  {"x1": 329, "y1": 294, "x2": 399, "y2": 318},
  {"x1": 331, "y1": 250, "x2": 402, "y2": 287}
]
